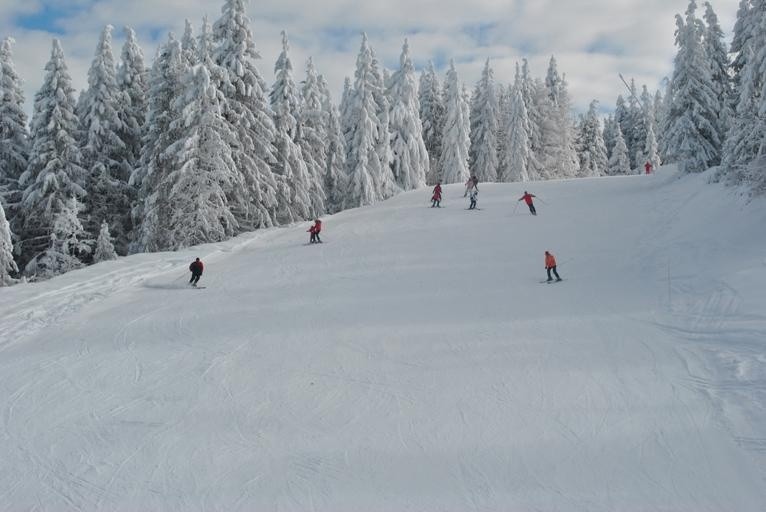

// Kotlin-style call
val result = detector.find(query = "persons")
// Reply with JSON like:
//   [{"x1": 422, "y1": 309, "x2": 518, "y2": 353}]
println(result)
[
  {"x1": 463, "y1": 177, "x2": 473, "y2": 198},
  {"x1": 432, "y1": 182, "x2": 442, "y2": 194},
  {"x1": 314, "y1": 219, "x2": 322, "y2": 243},
  {"x1": 188, "y1": 258, "x2": 204, "y2": 287},
  {"x1": 645, "y1": 161, "x2": 651, "y2": 175},
  {"x1": 472, "y1": 175, "x2": 479, "y2": 190},
  {"x1": 430, "y1": 189, "x2": 441, "y2": 208},
  {"x1": 306, "y1": 225, "x2": 316, "y2": 244},
  {"x1": 469, "y1": 191, "x2": 478, "y2": 209},
  {"x1": 544, "y1": 250, "x2": 561, "y2": 281},
  {"x1": 519, "y1": 191, "x2": 536, "y2": 215}
]
